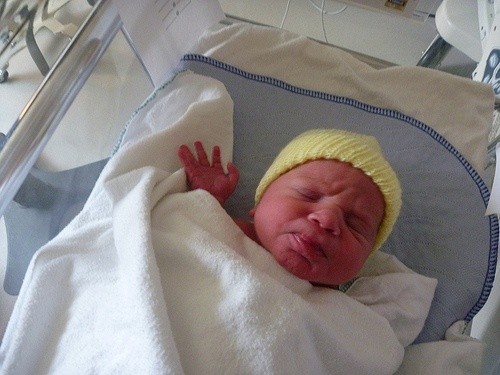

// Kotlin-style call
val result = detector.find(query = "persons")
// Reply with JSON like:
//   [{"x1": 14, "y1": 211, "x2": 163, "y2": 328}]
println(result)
[{"x1": 47, "y1": 126, "x2": 406, "y2": 375}]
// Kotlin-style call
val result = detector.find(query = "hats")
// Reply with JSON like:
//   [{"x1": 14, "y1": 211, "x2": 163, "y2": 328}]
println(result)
[{"x1": 253, "y1": 128, "x2": 402, "y2": 267}]
